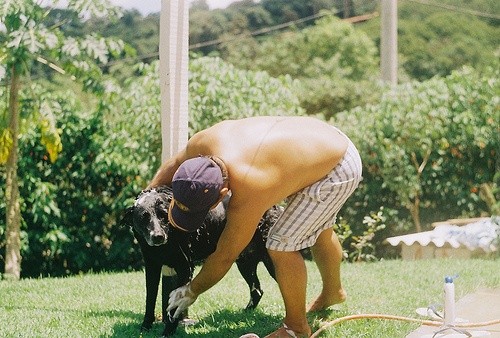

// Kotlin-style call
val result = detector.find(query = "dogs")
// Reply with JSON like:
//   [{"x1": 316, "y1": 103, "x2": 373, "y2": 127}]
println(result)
[{"x1": 118, "y1": 184, "x2": 284, "y2": 337}]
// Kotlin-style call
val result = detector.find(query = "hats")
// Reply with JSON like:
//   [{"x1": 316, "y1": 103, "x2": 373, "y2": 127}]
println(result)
[{"x1": 170, "y1": 157, "x2": 224, "y2": 232}]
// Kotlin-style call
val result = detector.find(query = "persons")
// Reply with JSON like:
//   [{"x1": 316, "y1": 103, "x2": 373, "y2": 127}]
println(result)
[{"x1": 142, "y1": 116, "x2": 363, "y2": 338}]
